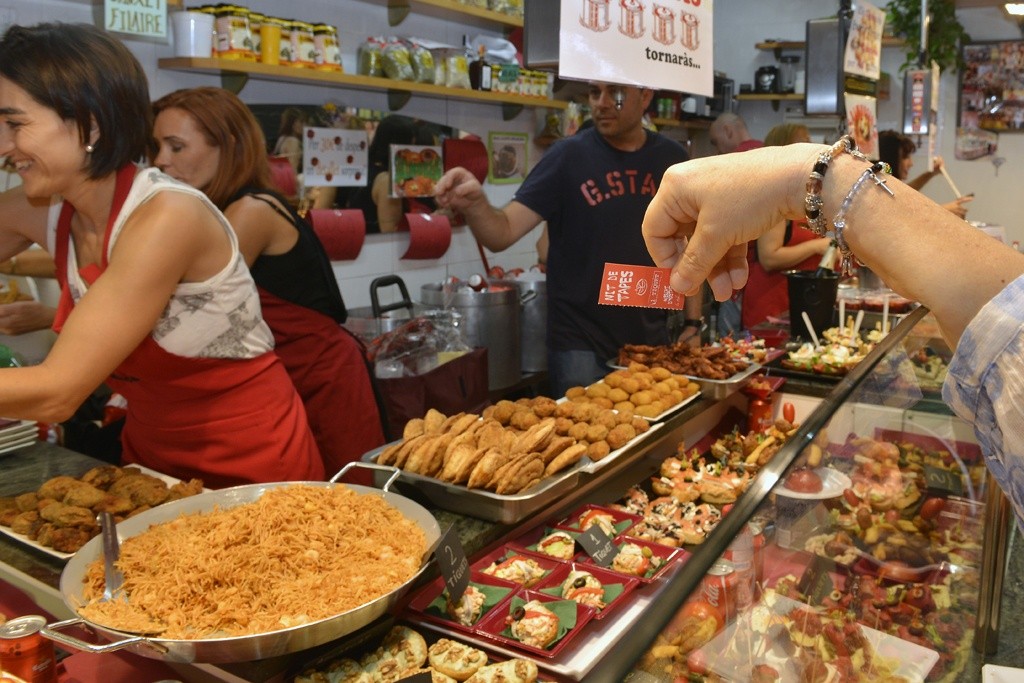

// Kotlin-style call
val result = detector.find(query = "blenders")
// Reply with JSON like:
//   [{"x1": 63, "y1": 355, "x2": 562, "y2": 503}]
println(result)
[{"x1": 779, "y1": 55, "x2": 802, "y2": 93}]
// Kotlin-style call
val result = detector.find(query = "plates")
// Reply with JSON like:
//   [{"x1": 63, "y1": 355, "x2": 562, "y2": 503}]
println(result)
[
  {"x1": 690, "y1": 592, "x2": 941, "y2": 682},
  {"x1": 0, "y1": 461, "x2": 214, "y2": 560},
  {"x1": 0, "y1": 421, "x2": 39, "y2": 453},
  {"x1": 480, "y1": 375, "x2": 703, "y2": 471},
  {"x1": 770, "y1": 466, "x2": 852, "y2": 501}
]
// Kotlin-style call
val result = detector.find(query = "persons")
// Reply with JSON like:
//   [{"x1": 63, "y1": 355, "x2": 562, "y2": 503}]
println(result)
[
  {"x1": 146, "y1": 83, "x2": 392, "y2": 489},
  {"x1": 643, "y1": 144, "x2": 1024, "y2": 529},
  {"x1": 712, "y1": 115, "x2": 974, "y2": 353},
  {"x1": 436, "y1": 77, "x2": 695, "y2": 393},
  {"x1": 0, "y1": 20, "x2": 325, "y2": 494},
  {"x1": 276, "y1": 106, "x2": 382, "y2": 191},
  {"x1": 962, "y1": 42, "x2": 1024, "y2": 130}
]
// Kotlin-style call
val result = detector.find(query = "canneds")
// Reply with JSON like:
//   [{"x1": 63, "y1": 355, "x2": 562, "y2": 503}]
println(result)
[
  {"x1": 186, "y1": 2, "x2": 343, "y2": 70},
  {"x1": 442, "y1": 273, "x2": 513, "y2": 293},
  {"x1": 489, "y1": 264, "x2": 545, "y2": 280},
  {"x1": 703, "y1": 558, "x2": 738, "y2": 630},
  {"x1": 725, "y1": 521, "x2": 766, "y2": 613},
  {"x1": 750, "y1": 397, "x2": 773, "y2": 434},
  {"x1": 0, "y1": 614, "x2": 58, "y2": 683},
  {"x1": 490, "y1": 65, "x2": 547, "y2": 97}
]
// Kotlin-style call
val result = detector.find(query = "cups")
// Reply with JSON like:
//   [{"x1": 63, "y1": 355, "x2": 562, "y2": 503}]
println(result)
[
  {"x1": 170, "y1": 11, "x2": 216, "y2": 58},
  {"x1": 705, "y1": 105, "x2": 711, "y2": 116},
  {"x1": 846, "y1": 289, "x2": 915, "y2": 313},
  {"x1": 258, "y1": 22, "x2": 282, "y2": 65},
  {"x1": 680, "y1": 97, "x2": 697, "y2": 114}
]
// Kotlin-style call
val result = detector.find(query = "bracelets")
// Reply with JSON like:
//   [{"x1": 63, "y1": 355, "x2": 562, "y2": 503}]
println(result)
[
  {"x1": 7, "y1": 256, "x2": 17, "y2": 274},
  {"x1": 805, "y1": 133, "x2": 865, "y2": 237},
  {"x1": 832, "y1": 162, "x2": 896, "y2": 269}
]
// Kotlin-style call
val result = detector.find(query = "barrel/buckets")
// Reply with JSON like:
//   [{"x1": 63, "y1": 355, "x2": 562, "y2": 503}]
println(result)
[
  {"x1": 783, "y1": 269, "x2": 839, "y2": 342},
  {"x1": 340, "y1": 268, "x2": 548, "y2": 393}
]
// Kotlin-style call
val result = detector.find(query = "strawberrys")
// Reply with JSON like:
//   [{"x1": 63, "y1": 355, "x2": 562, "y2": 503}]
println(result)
[{"x1": 366, "y1": 315, "x2": 434, "y2": 363}]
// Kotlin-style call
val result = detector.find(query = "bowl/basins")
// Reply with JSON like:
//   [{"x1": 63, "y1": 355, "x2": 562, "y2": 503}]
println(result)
[
  {"x1": 750, "y1": 329, "x2": 791, "y2": 347},
  {"x1": 409, "y1": 503, "x2": 693, "y2": 660}
]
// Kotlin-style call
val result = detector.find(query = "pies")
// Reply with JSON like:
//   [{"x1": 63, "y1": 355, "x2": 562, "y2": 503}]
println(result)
[{"x1": 376, "y1": 406, "x2": 588, "y2": 494}]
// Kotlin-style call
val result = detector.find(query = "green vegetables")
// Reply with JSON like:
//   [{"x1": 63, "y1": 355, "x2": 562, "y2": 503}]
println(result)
[{"x1": 787, "y1": 349, "x2": 844, "y2": 374}]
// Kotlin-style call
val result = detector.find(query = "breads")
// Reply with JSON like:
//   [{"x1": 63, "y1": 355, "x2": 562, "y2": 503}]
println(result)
[{"x1": 481, "y1": 363, "x2": 700, "y2": 459}]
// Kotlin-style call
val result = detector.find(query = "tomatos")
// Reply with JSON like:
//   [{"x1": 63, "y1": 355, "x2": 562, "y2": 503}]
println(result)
[
  {"x1": 673, "y1": 648, "x2": 708, "y2": 683},
  {"x1": 398, "y1": 148, "x2": 440, "y2": 195}
]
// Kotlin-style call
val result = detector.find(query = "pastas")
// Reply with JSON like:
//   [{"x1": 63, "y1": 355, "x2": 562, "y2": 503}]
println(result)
[{"x1": 71, "y1": 483, "x2": 428, "y2": 640}]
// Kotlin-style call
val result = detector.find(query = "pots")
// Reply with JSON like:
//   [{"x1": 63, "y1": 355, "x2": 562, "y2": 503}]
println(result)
[
  {"x1": 969, "y1": 219, "x2": 1007, "y2": 245},
  {"x1": 38, "y1": 457, "x2": 441, "y2": 654}
]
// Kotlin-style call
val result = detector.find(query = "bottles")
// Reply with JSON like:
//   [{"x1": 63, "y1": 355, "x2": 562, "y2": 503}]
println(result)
[
  {"x1": 812, "y1": 239, "x2": 838, "y2": 277},
  {"x1": 1012, "y1": 240, "x2": 1019, "y2": 252},
  {"x1": 657, "y1": 98, "x2": 673, "y2": 119},
  {"x1": 545, "y1": 106, "x2": 560, "y2": 137}
]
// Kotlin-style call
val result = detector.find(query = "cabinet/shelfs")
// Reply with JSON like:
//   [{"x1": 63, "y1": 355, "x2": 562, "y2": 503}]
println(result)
[
  {"x1": 159, "y1": 0, "x2": 710, "y2": 131},
  {"x1": 736, "y1": 42, "x2": 805, "y2": 112}
]
